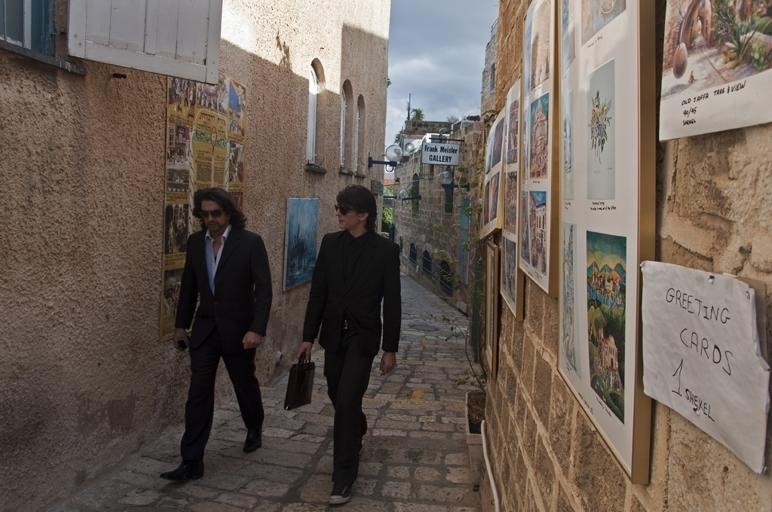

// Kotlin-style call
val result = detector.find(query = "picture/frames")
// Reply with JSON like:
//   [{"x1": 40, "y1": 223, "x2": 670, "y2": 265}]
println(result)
[
  {"x1": 556, "y1": 0, "x2": 659, "y2": 486},
  {"x1": 519, "y1": 0, "x2": 554, "y2": 296},
  {"x1": 477, "y1": 76, "x2": 526, "y2": 385}
]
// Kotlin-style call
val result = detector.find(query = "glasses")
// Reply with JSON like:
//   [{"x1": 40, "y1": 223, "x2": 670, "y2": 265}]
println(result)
[
  {"x1": 335, "y1": 205, "x2": 354, "y2": 215},
  {"x1": 201, "y1": 210, "x2": 222, "y2": 216}
]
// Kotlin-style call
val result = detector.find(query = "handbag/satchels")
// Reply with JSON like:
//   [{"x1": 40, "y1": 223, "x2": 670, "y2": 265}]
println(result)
[{"x1": 284, "y1": 350, "x2": 315, "y2": 411}]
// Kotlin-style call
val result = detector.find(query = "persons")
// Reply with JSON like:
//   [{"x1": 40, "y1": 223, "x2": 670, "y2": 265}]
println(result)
[
  {"x1": 159, "y1": 188, "x2": 273, "y2": 480},
  {"x1": 296, "y1": 185, "x2": 402, "y2": 505}
]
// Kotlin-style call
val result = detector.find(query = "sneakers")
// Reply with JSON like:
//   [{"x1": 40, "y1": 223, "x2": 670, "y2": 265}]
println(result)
[{"x1": 329, "y1": 480, "x2": 352, "y2": 504}]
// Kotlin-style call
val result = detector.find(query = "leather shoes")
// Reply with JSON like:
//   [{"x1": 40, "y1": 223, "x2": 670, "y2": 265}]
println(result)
[
  {"x1": 160, "y1": 459, "x2": 204, "y2": 480},
  {"x1": 243, "y1": 426, "x2": 261, "y2": 453}
]
